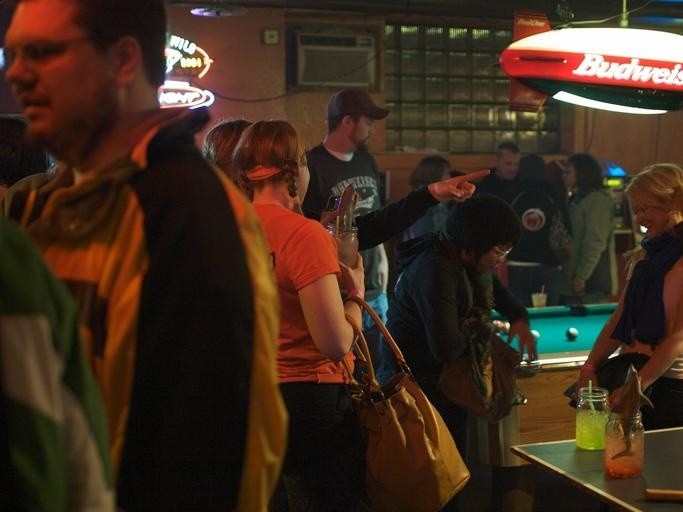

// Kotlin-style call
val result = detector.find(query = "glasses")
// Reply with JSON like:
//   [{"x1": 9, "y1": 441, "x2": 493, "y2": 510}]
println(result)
[{"x1": 492, "y1": 245, "x2": 513, "y2": 257}]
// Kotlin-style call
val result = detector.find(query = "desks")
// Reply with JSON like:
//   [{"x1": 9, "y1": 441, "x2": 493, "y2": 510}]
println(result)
[
  {"x1": 491, "y1": 303, "x2": 622, "y2": 467},
  {"x1": 510, "y1": 427, "x2": 682, "y2": 512}
]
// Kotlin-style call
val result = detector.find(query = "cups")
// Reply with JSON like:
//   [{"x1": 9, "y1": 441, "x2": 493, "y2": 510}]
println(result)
[
  {"x1": 324, "y1": 223, "x2": 359, "y2": 289},
  {"x1": 604, "y1": 409, "x2": 645, "y2": 479},
  {"x1": 575, "y1": 387, "x2": 609, "y2": 450},
  {"x1": 531, "y1": 293, "x2": 547, "y2": 308}
]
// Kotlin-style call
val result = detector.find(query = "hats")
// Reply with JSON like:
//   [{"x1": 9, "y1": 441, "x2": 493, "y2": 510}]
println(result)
[{"x1": 328, "y1": 88, "x2": 390, "y2": 120}]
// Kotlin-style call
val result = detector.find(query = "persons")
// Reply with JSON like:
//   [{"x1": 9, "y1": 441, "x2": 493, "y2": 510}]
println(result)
[
  {"x1": 200, "y1": 88, "x2": 618, "y2": 509},
  {"x1": 0, "y1": 204, "x2": 118, "y2": 512},
  {"x1": 2, "y1": 1, "x2": 290, "y2": 512},
  {"x1": 571, "y1": 163, "x2": 683, "y2": 433}
]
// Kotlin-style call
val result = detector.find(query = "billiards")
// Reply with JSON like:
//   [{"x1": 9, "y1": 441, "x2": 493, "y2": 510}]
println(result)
[
  {"x1": 503, "y1": 322, "x2": 510, "y2": 332},
  {"x1": 530, "y1": 330, "x2": 539, "y2": 342},
  {"x1": 566, "y1": 329, "x2": 577, "y2": 340},
  {"x1": 493, "y1": 319, "x2": 502, "y2": 330}
]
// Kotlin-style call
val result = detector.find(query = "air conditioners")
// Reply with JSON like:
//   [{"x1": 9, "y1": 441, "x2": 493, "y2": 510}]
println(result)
[{"x1": 295, "y1": 29, "x2": 377, "y2": 88}]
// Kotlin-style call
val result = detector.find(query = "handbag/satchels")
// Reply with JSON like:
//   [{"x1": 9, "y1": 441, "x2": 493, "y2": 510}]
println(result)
[
  {"x1": 349, "y1": 370, "x2": 470, "y2": 511},
  {"x1": 549, "y1": 209, "x2": 572, "y2": 263},
  {"x1": 437, "y1": 334, "x2": 522, "y2": 420},
  {"x1": 566, "y1": 353, "x2": 655, "y2": 415}
]
